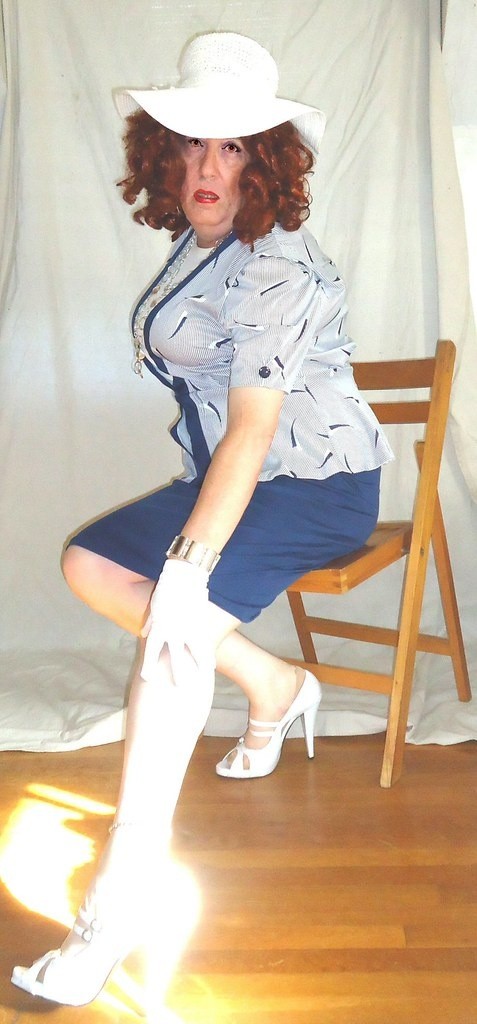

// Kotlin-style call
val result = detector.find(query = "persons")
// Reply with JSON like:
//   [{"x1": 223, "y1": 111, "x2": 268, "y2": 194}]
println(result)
[{"x1": 12, "y1": 28, "x2": 386, "y2": 1008}]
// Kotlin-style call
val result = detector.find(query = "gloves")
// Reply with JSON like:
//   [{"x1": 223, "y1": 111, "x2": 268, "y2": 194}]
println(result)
[{"x1": 139, "y1": 558, "x2": 214, "y2": 688}]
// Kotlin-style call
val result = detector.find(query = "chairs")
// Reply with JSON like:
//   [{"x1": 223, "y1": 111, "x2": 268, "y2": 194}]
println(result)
[{"x1": 280, "y1": 341, "x2": 471, "y2": 790}]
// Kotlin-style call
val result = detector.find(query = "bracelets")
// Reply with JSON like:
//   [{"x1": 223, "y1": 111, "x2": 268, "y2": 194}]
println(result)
[{"x1": 164, "y1": 534, "x2": 221, "y2": 573}]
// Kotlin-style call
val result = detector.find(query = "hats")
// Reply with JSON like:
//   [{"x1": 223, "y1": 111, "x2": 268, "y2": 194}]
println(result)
[{"x1": 114, "y1": 33, "x2": 327, "y2": 161}]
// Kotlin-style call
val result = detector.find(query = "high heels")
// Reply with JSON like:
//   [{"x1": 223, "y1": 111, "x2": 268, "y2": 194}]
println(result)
[
  {"x1": 14, "y1": 864, "x2": 203, "y2": 1007},
  {"x1": 214, "y1": 667, "x2": 321, "y2": 778}
]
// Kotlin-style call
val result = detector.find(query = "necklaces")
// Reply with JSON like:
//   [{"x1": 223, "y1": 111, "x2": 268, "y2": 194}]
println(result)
[{"x1": 132, "y1": 229, "x2": 235, "y2": 378}]
